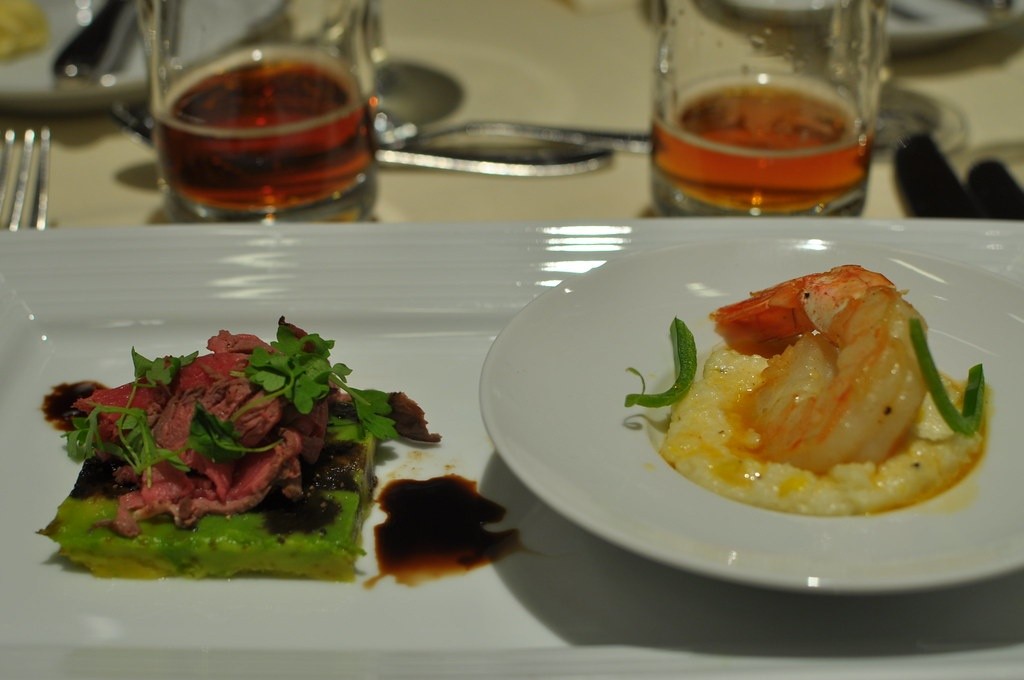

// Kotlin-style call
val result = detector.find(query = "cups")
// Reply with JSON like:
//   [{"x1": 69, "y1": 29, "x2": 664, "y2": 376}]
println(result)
[
  {"x1": 146, "y1": 0, "x2": 377, "y2": 223},
  {"x1": 647, "y1": 0, "x2": 894, "y2": 220}
]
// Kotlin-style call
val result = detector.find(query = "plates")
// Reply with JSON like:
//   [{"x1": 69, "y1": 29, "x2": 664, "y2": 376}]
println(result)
[
  {"x1": 0, "y1": 0, "x2": 310, "y2": 118},
  {"x1": 698, "y1": 0, "x2": 1024, "y2": 56},
  {"x1": 0, "y1": 216, "x2": 1024, "y2": 680},
  {"x1": 478, "y1": 234, "x2": 1022, "y2": 591}
]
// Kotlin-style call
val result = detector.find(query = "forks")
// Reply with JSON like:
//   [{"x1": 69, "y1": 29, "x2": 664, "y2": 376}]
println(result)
[{"x1": 0, "y1": 127, "x2": 60, "y2": 234}]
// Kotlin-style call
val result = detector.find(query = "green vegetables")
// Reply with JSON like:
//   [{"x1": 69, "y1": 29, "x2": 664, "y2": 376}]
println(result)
[{"x1": 60, "y1": 327, "x2": 399, "y2": 486}]
